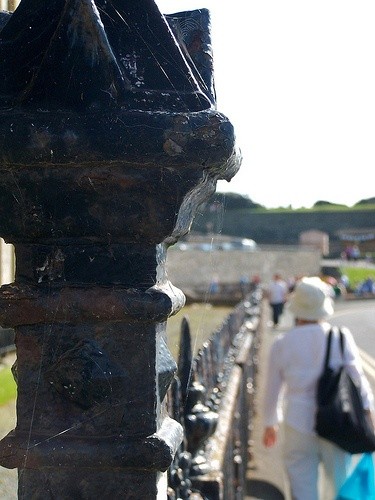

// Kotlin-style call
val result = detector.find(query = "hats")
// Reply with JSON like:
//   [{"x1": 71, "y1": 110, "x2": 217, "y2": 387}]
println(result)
[{"x1": 287, "y1": 276, "x2": 335, "y2": 321}]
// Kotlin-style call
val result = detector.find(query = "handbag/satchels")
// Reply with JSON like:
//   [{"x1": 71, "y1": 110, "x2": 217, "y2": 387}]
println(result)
[{"x1": 314, "y1": 325, "x2": 375, "y2": 454}]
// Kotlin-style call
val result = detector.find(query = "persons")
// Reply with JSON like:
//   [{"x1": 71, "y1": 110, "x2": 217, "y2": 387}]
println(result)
[
  {"x1": 327, "y1": 274, "x2": 375, "y2": 295},
  {"x1": 262, "y1": 276, "x2": 375, "y2": 500},
  {"x1": 268, "y1": 273, "x2": 286, "y2": 327}
]
